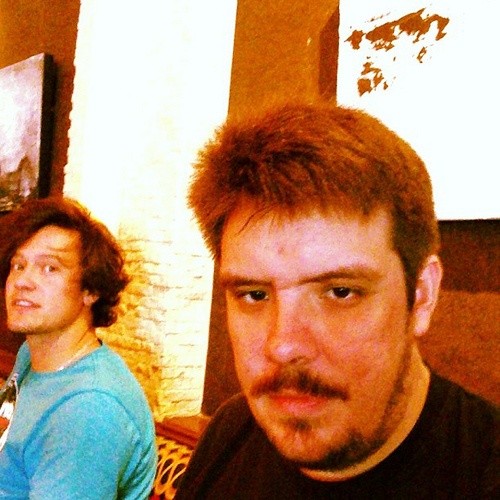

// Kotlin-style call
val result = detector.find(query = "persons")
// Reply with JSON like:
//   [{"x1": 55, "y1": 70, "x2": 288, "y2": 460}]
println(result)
[
  {"x1": 0, "y1": 197, "x2": 158, "y2": 500},
  {"x1": 173, "y1": 101, "x2": 500, "y2": 500}
]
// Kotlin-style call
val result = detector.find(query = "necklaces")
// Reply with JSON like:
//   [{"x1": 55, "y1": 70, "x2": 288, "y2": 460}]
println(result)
[{"x1": 57, "y1": 337, "x2": 99, "y2": 371}]
